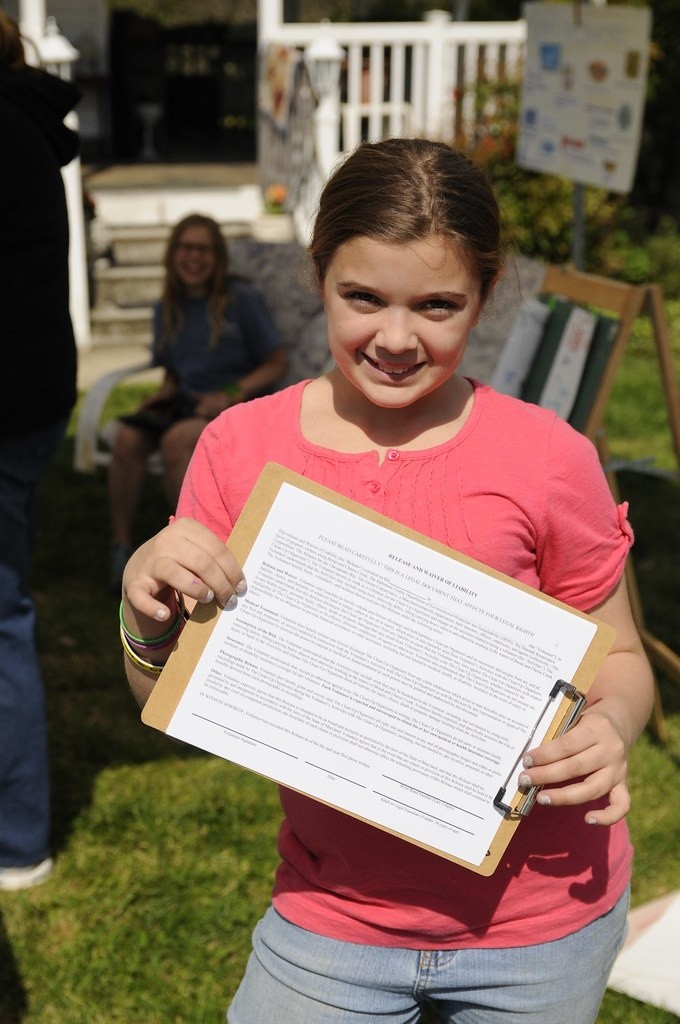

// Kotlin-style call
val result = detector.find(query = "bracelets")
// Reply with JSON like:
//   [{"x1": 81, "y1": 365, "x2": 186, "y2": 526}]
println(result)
[{"x1": 119, "y1": 590, "x2": 191, "y2": 673}]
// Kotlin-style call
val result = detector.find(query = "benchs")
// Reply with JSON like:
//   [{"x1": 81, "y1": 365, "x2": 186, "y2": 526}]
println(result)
[{"x1": 70, "y1": 239, "x2": 550, "y2": 497}]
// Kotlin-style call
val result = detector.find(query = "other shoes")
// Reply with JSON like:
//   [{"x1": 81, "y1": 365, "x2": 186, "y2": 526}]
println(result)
[
  {"x1": 0, "y1": 853, "x2": 52, "y2": 891},
  {"x1": 104, "y1": 544, "x2": 136, "y2": 594}
]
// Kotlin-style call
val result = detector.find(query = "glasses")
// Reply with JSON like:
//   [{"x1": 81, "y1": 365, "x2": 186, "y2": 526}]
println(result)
[{"x1": 177, "y1": 242, "x2": 220, "y2": 253}]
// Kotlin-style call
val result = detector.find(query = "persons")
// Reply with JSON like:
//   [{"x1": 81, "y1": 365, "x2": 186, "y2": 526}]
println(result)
[
  {"x1": 109, "y1": 215, "x2": 287, "y2": 585},
  {"x1": 0, "y1": 11, "x2": 82, "y2": 889},
  {"x1": 121, "y1": 138, "x2": 654, "y2": 1024}
]
